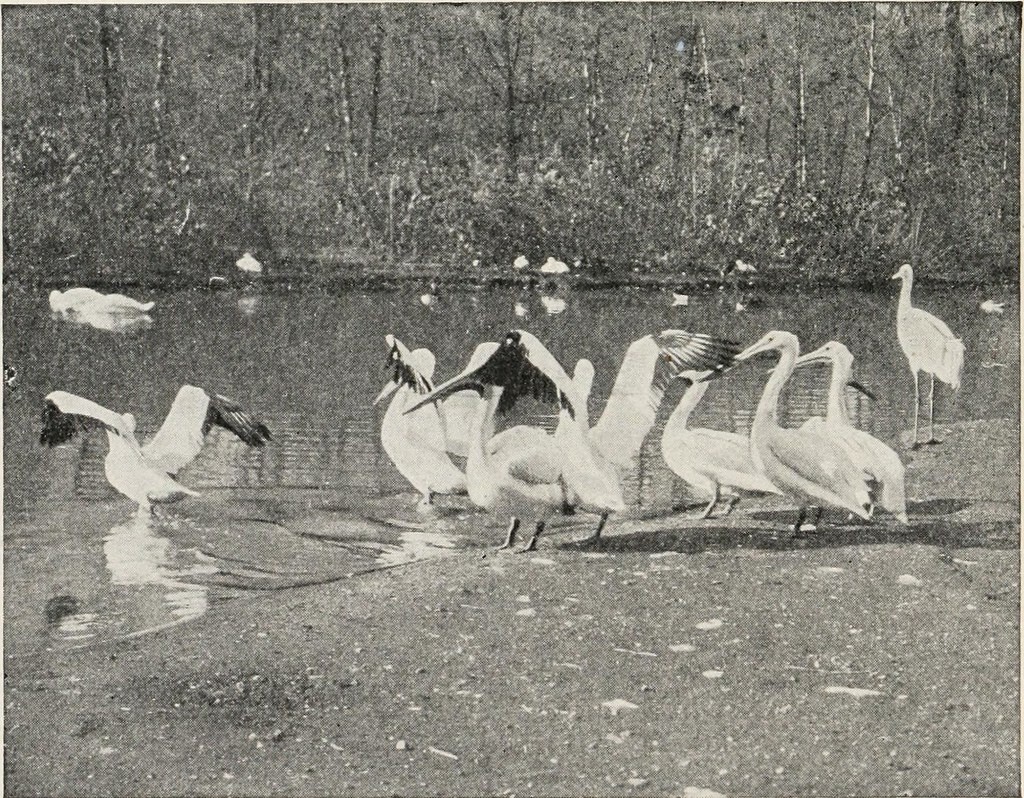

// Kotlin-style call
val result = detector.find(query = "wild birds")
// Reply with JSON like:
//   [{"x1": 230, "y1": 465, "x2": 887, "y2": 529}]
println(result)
[
  {"x1": 35, "y1": 384, "x2": 275, "y2": 519},
  {"x1": 401, "y1": 362, "x2": 579, "y2": 555},
  {"x1": 890, "y1": 264, "x2": 967, "y2": 448},
  {"x1": 372, "y1": 334, "x2": 501, "y2": 506},
  {"x1": 467, "y1": 329, "x2": 742, "y2": 549},
  {"x1": 764, "y1": 340, "x2": 912, "y2": 529},
  {"x1": 660, "y1": 369, "x2": 786, "y2": 521},
  {"x1": 697, "y1": 331, "x2": 885, "y2": 538}
]
